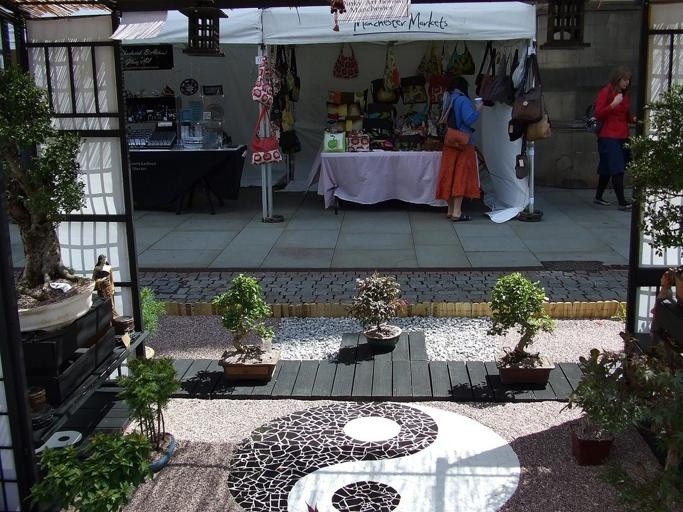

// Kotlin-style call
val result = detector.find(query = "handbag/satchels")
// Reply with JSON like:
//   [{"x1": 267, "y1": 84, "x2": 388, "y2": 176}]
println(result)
[
  {"x1": 446, "y1": 41, "x2": 476, "y2": 76},
  {"x1": 417, "y1": 43, "x2": 445, "y2": 76},
  {"x1": 323, "y1": 75, "x2": 451, "y2": 153},
  {"x1": 508, "y1": 53, "x2": 550, "y2": 141},
  {"x1": 475, "y1": 41, "x2": 520, "y2": 106},
  {"x1": 272, "y1": 44, "x2": 302, "y2": 153},
  {"x1": 333, "y1": 42, "x2": 360, "y2": 79},
  {"x1": 384, "y1": 44, "x2": 400, "y2": 91},
  {"x1": 251, "y1": 107, "x2": 283, "y2": 165},
  {"x1": 514, "y1": 154, "x2": 530, "y2": 179},
  {"x1": 444, "y1": 128, "x2": 470, "y2": 152},
  {"x1": 252, "y1": 44, "x2": 273, "y2": 107}
]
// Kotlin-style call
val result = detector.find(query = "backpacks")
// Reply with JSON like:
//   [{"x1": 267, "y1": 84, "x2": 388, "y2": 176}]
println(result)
[{"x1": 584, "y1": 104, "x2": 604, "y2": 132}]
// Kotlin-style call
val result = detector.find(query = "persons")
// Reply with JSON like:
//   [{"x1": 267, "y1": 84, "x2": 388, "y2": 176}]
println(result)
[
  {"x1": 594, "y1": 65, "x2": 637, "y2": 210},
  {"x1": 92, "y1": 255, "x2": 115, "y2": 311},
  {"x1": 434, "y1": 77, "x2": 484, "y2": 221}
]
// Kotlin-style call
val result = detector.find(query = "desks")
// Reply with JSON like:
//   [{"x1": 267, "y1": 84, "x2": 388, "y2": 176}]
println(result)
[
  {"x1": 127, "y1": 144, "x2": 248, "y2": 215},
  {"x1": 317, "y1": 148, "x2": 480, "y2": 215}
]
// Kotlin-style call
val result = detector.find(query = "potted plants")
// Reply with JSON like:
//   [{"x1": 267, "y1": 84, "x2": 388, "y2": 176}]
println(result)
[
  {"x1": 344, "y1": 271, "x2": 402, "y2": 354},
  {"x1": 486, "y1": 272, "x2": 556, "y2": 390},
  {"x1": 568, "y1": 352, "x2": 673, "y2": 466},
  {"x1": 115, "y1": 358, "x2": 181, "y2": 473},
  {"x1": 210, "y1": 274, "x2": 278, "y2": 386},
  {"x1": 1, "y1": 69, "x2": 96, "y2": 333}
]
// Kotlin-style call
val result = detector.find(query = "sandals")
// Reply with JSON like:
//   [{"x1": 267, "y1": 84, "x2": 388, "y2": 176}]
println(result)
[
  {"x1": 619, "y1": 203, "x2": 632, "y2": 210},
  {"x1": 446, "y1": 214, "x2": 472, "y2": 221},
  {"x1": 593, "y1": 197, "x2": 612, "y2": 206}
]
